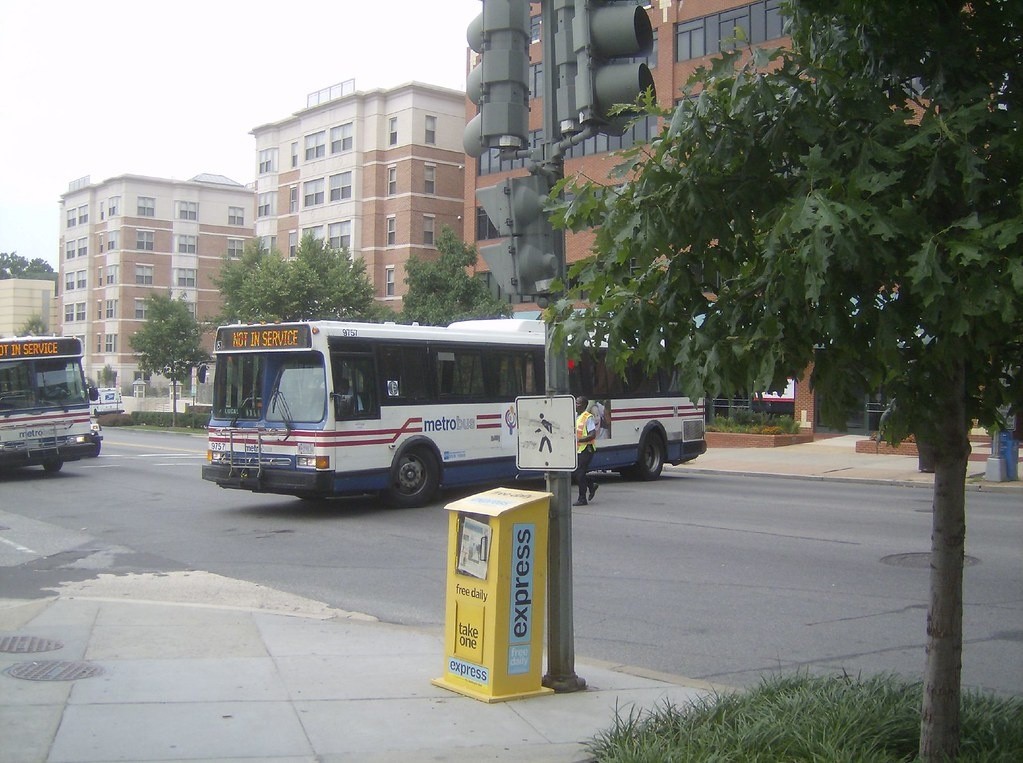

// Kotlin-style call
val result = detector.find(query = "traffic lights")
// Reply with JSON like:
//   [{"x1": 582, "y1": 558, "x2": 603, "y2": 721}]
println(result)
[
  {"x1": 473, "y1": 172, "x2": 555, "y2": 298},
  {"x1": 569, "y1": 0, "x2": 656, "y2": 134},
  {"x1": 464, "y1": 0, "x2": 528, "y2": 160}
]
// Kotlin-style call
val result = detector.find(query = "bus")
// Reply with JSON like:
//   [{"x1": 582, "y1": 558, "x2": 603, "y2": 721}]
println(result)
[
  {"x1": 202, "y1": 318, "x2": 708, "y2": 509},
  {"x1": 0, "y1": 335, "x2": 98, "y2": 471}
]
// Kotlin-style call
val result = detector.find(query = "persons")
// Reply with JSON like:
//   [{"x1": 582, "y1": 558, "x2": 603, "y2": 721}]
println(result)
[
  {"x1": 336, "y1": 377, "x2": 363, "y2": 415},
  {"x1": 573, "y1": 395, "x2": 599, "y2": 506},
  {"x1": 590, "y1": 405, "x2": 611, "y2": 439},
  {"x1": 43, "y1": 375, "x2": 70, "y2": 400}
]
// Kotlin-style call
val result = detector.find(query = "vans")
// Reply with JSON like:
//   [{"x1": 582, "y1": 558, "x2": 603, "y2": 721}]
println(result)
[{"x1": 88, "y1": 388, "x2": 125, "y2": 416}]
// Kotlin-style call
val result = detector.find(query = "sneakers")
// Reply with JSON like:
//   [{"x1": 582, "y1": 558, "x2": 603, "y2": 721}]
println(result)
[
  {"x1": 573, "y1": 501, "x2": 588, "y2": 506},
  {"x1": 588, "y1": 483, "x2": 599, "y2": 501}
]
